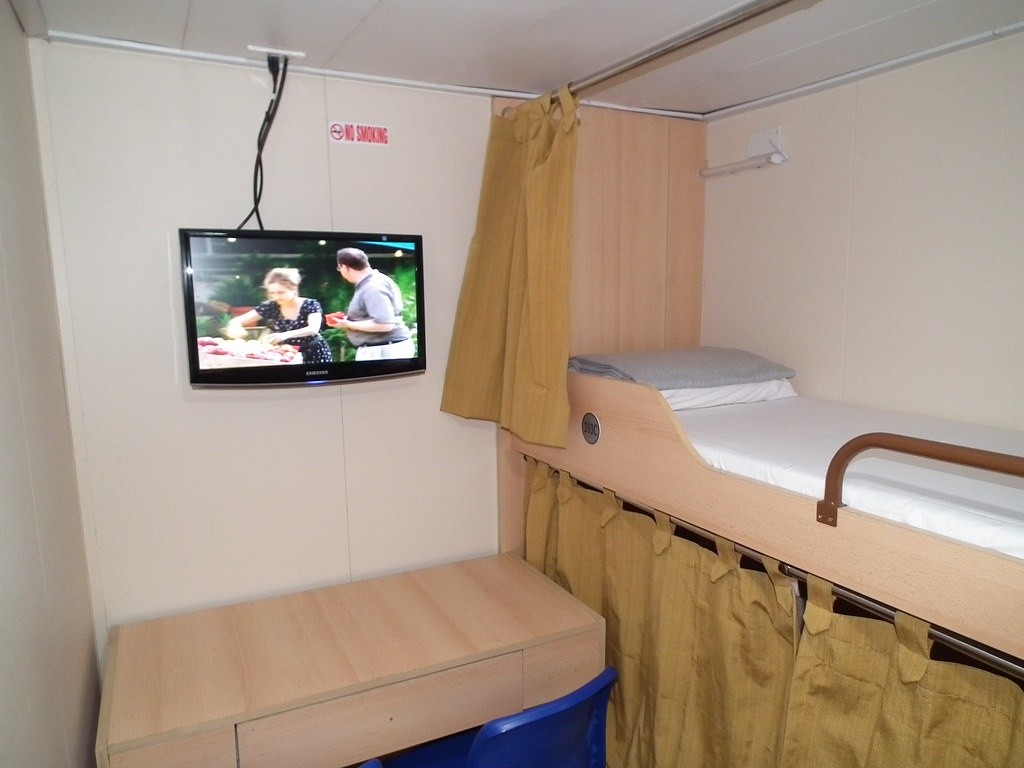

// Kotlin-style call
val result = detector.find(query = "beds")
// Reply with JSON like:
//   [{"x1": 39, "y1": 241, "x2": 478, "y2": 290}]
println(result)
[{"x1": 498, "y1": 373, "x2": 1024, "y2": 661}]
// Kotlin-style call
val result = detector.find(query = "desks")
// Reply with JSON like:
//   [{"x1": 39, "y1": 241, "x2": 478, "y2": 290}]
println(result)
[{"x1": 95, "y1": 550, "x2": 606, "y2": 768}]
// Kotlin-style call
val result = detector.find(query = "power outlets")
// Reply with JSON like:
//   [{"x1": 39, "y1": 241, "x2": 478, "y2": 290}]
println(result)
[{"x1": 247, "y1": 45, "x2": 305, "y2": 66}]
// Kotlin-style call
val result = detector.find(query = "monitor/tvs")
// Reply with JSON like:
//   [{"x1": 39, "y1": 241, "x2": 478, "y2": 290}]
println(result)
[{"x1": 179, "y1": 228, "x2": 426, "y2": 386}]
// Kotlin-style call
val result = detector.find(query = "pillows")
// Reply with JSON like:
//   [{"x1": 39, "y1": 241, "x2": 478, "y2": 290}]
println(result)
[{"x1": 660, "y1": 378, "x2": 797, "y2": 411}]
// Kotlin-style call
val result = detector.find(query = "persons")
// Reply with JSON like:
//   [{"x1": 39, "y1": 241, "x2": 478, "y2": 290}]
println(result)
[
  {"x1": 327, "y1": 247, "x2": 416, "y2": 359},
  {"x1": 228, "y1": 267, "x2": 333, "y2": 363}
]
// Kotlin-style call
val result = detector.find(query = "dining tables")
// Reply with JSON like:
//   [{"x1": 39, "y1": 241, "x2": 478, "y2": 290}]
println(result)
[{"x1": 198, "y1": 340, "x2": 303, "y2": 369}]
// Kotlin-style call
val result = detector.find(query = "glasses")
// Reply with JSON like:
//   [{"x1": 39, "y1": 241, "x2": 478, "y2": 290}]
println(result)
[{"x1": 336, "y1": 264, "x2": 352, "y2": 272}]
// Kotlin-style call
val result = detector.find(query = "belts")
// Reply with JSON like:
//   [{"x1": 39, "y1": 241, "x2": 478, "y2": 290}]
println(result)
[{"x1": 359, "y1": 338, "x2": 411, "y2": 346}]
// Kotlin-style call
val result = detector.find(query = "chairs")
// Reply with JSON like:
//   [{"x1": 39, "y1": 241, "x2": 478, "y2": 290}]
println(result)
[{"x1": 357, "y1": 668, "x2": 619, "y2": 768}]
